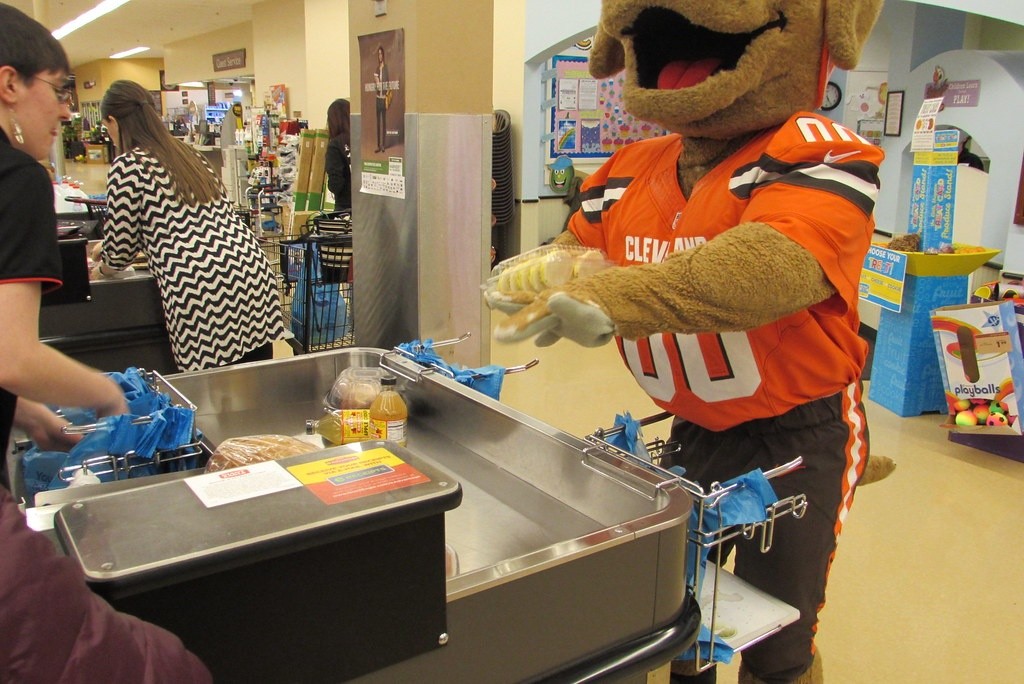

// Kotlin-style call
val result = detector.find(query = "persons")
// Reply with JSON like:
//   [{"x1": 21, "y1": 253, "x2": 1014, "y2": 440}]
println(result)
[
  {"x1": 177, "y1": 118, "x2": 186, "y2": 131},
  {"x1": 374, "y1": 46, "x2": 392, "y2": 153},
  {"x1": 0, "y1": 487, "x2": 215, "y2": 684},
  {"x1": 90, "y1": 80, "x2": 285, "y2": 373},
  {"x1": 94, "y1": 120, "x2": 114, "y2": 163},
  {"x1": 0, "y1": 5, "x2": 129, "y2": 481},
  {"x1": 326, "y1": 99, "x2": 352, "y2": 210}
]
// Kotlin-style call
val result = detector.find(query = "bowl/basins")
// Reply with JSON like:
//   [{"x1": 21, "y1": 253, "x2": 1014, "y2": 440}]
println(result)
[{"x1": 321, "y1": 365, "x2": 410, "y2": 415}]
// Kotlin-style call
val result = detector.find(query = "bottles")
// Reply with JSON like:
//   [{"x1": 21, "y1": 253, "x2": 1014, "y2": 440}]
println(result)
[
  {"x1": 369, "y1": 374, "x2": 407, "y2": 448},
  {"x1": 306, "y1": 409, "x2": 370, "y2": 446},
  {"x1": 52, "y1": 179, "x2": 88, "y2": 213}
]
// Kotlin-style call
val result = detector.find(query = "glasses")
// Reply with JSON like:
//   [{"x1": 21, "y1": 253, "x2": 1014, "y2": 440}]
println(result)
[{"x1": 15, "y1": 68, "x2": 76, "y2": 107}]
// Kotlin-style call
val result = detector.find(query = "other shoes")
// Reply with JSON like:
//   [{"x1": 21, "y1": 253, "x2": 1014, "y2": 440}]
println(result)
[
  {"x1": 382, "y1": 147, "x2": 385, "y2": 152},
  {"x1": 375, "y1": 148, "x2": 381, "y2": 153}
]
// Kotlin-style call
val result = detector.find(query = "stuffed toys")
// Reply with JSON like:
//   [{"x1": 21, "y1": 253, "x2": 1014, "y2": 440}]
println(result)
[{"x1": 484, "y1": 0, "x2": 884, "y2": 684}]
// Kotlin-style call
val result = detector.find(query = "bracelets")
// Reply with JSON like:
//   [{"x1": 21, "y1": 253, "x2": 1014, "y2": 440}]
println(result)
[{"x1": 98, "y1": 265, "x2": 110, "y2": 278}]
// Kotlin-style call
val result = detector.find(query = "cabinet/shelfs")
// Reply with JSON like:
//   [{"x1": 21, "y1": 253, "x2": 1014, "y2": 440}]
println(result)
[{"x1": 203, "y1": 104, "x2": 228, "y2": 124}]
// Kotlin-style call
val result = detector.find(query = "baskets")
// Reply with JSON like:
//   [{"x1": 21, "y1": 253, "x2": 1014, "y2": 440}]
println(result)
[{"x1": 492, "y1": 109, "x2": 514, "y2": 266}]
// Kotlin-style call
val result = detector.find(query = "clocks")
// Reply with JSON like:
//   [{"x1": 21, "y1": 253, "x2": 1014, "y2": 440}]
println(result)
[{"x1": 821, "y1": 81, "x2": 842, "y2": 112}]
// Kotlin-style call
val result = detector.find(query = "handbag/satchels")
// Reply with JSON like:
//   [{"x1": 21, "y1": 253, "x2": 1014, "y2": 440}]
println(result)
[
  {"x1": 385, "y1": 89, "x2": 393, "y2": 110},
  {"x1": 290, "y1": 242, "x2": 347, "y2": 346}
]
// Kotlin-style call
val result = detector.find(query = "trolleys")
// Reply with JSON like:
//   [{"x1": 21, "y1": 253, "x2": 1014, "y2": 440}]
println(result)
[
  {"x1": 65, "y1": 195, "x2": 268, "y2": 244},
  {"x1": 257, "y1": 210, "x2": 358, "y2": 358}
]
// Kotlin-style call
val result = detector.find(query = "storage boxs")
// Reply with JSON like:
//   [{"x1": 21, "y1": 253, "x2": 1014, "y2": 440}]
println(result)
[
  {"x1": 287, "y1": 126, "x2": 330, "y2": 240},
  {"x1": 930, "y1": 297, "x2": 1024, "y2": 438}
]
